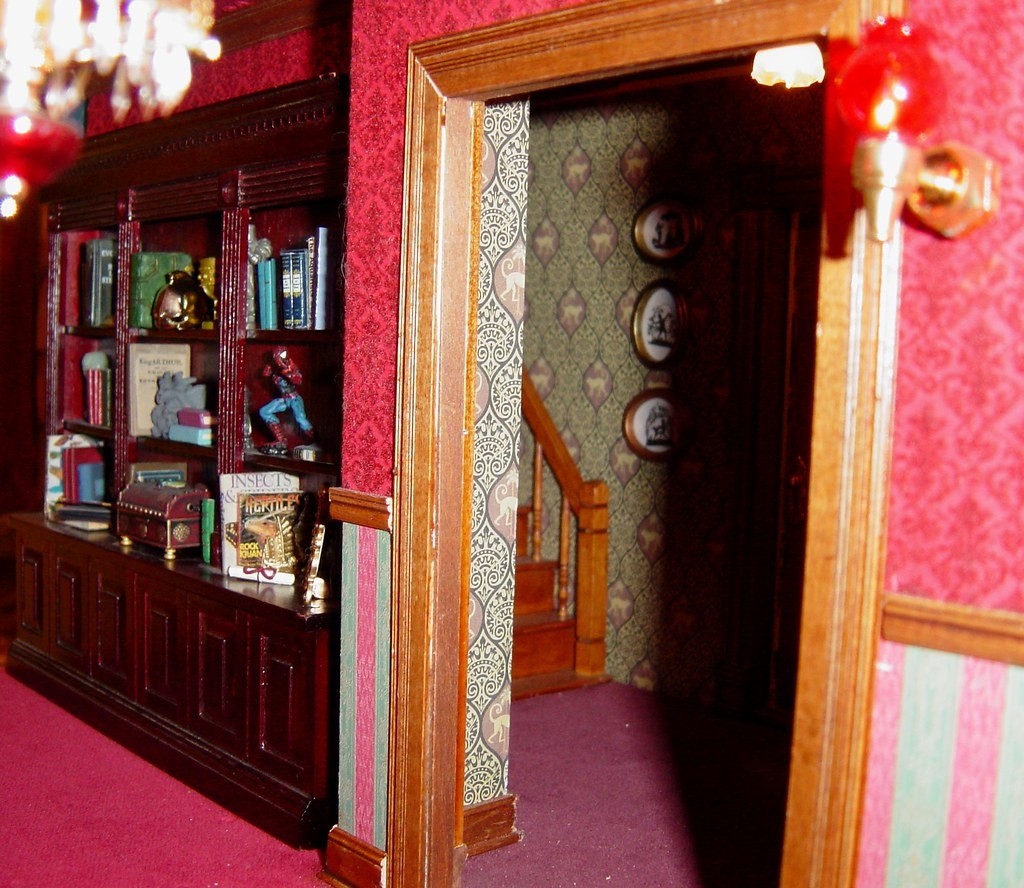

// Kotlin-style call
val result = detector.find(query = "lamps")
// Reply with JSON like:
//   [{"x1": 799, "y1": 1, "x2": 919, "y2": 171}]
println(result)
[
  {"x1": 835, "y1": 15, "x2": 1002, "y2": 243},
  {"x1": 751, "y1": 42, "x2": 825, "y2": 90},
  {"x1": 0, "y1": 0, "x2": 222, "y2": 223}
]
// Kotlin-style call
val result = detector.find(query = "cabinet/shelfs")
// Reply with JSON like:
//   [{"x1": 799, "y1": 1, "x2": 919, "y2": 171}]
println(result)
[{"x1": 0, "y1": 71, "x2": 346, "y2": 849}]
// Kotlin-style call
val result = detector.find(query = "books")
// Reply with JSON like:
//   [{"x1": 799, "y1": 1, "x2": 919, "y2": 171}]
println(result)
[{"x1": 49, "y1": 222, "x2": 343, "y2": 592}]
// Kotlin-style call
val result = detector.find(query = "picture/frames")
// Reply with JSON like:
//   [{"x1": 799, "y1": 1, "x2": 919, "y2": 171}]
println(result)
[
  {"x1": 623, "y1": 386, "x2": 681, "y2": 462},
  {"x1": 632, "y1": 201, "x2": 694, "y2": 261},
  {"x1": 629, "y1": 282, "x2": 678, "y2": 365}
]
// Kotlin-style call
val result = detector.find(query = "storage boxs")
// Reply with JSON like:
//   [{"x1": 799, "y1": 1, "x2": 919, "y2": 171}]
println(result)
[{"x1": 116, "y1": 475, "x2": 212, "y2": 561}]
